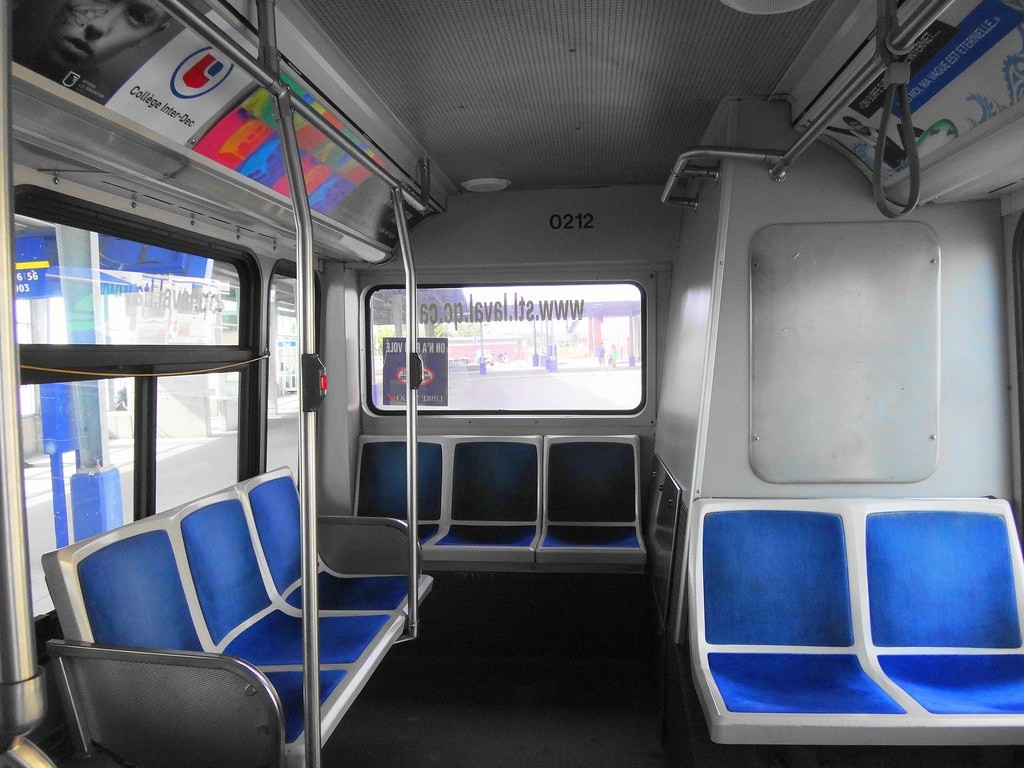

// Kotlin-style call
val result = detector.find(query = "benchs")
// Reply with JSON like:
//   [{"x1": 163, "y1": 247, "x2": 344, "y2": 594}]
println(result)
[
  {"x1": 687, "y1": 496, "x2": 1024, "y2": 768},
  {"x1": 40, "y1": 465, "x2": 433, "y2": 768},
  {"x1": 354, "y1": 433, "x2": 648, "y2": 575}
]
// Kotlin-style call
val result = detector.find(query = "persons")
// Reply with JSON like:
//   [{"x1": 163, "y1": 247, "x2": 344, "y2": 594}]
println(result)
[
  {"x1": 41, "y1": 0, "x2": 171, "y2": 87},
  {"x1": 597, "y1": 343, "x2": 606, "y2": 369},
  {"x1": 610, "y1": 344, "x2": 617, "y2": 368}
]
